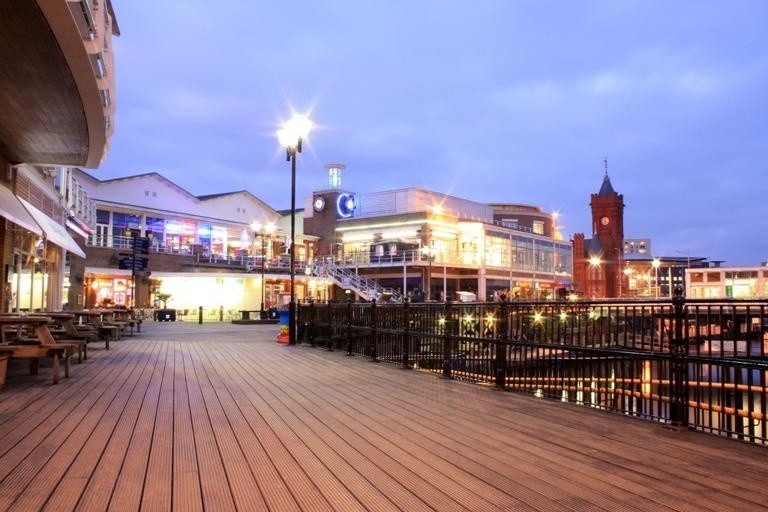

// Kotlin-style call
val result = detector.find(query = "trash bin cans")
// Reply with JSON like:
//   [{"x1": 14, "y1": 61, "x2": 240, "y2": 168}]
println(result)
[{"x1": 275, "y1": 309, "x2": 291, "y2": 325}]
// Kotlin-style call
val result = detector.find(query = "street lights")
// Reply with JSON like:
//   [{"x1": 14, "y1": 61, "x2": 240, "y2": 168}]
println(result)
[{"x1": 276, "y1": 112, "x2": 314, "y2": 347}]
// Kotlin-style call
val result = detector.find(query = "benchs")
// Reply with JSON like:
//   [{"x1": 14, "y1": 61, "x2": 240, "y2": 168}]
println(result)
[{"x1": 0, "y1": 318, "x2": 143, "y2": 385}]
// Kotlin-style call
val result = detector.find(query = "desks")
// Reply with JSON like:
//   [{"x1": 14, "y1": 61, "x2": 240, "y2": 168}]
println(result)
[{"x1": 0, "y1": 306, "x2": 133, "y2": 385}]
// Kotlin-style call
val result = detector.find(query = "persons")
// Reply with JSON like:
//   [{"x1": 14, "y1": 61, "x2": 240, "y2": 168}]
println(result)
[{"x1": 396, "y1": 286, "x2": 426, "y2": 302}]
